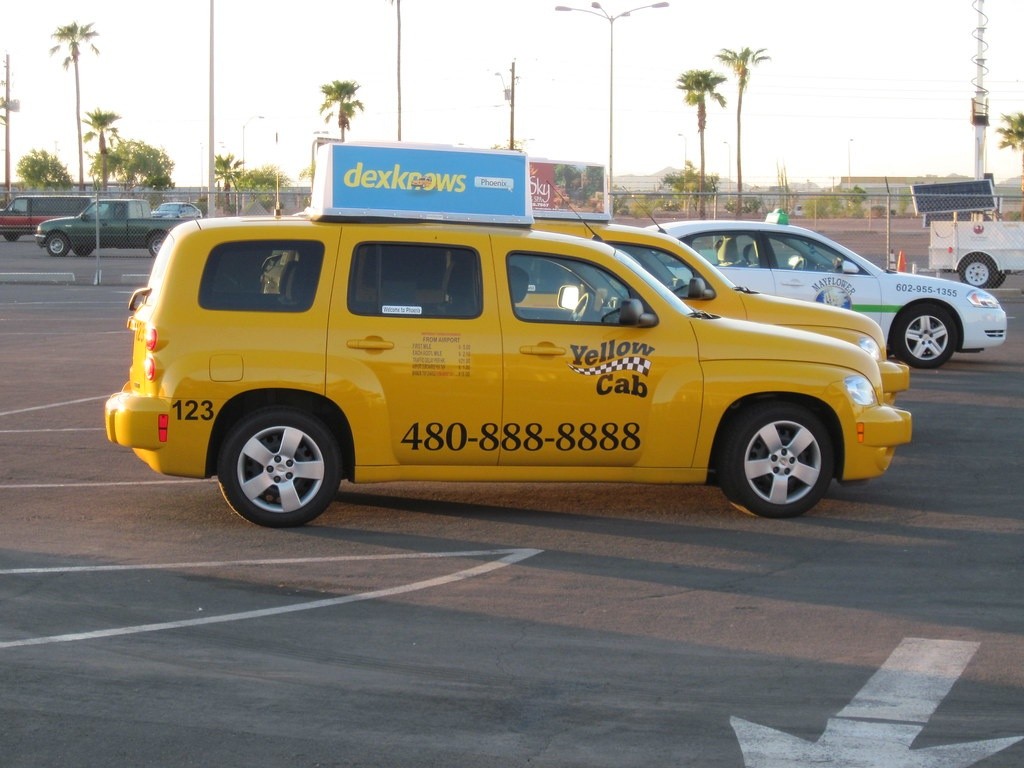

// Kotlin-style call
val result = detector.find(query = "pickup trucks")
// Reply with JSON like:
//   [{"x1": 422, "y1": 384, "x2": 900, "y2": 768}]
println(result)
[
  {"x1": 35, "y1": 198, "x2": 195, "y2": 258},
  {"x1": 927, "y1": 219, "x2": 1024, "y2": 289}
]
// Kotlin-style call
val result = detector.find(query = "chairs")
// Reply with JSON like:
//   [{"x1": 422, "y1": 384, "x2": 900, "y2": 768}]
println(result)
[
  {"x1": 743, "y1": 244, "x2": 758, "y2": 265},
  {"x1": 508, "y1": 265, "x2": 528, "y2": 303},
  {"x1": 434, "y1": 260, "x2": 477, "y2": 315},
  {"x1": 279, "y1": 261, "x2": 310, "y2": 303},
  {"x1": 718, "y1": 239, "x2": 739, "y2": 266}
]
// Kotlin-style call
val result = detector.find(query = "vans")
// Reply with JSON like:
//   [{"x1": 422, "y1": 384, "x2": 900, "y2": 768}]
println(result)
[{"x1": 0, "y1": 197, "x2": 107, "y2": 243}]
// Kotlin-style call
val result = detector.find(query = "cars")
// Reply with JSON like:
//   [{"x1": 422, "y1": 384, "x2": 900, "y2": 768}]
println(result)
[
  {"x1": 261, "y1": 151, "x2": 910, "y2": 408},
  {"x1": 102, "y1": 146, "x2": 913, "y2": 529},
  {"x1": 638, "y1": 208, "x2": 1008, "y2": 370},
  {"x1": 151, "y1": 202, "x2": 203, "y2": 220}
]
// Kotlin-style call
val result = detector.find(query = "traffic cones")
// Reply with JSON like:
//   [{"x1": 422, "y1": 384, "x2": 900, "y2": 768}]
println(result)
[
  {"x1": 890, "y1": 250, "x2": 896, "y2": 268},
  {"x1": 895, "y1": 250, "x2": 905, "y2": 270}
]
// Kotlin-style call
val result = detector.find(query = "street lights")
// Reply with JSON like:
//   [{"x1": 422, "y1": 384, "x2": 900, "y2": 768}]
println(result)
[
  {"x1": 200, "y1": 141, "x2": 227, "y2": 197},
  {"x1": 848, "y1": 139, "x2": 854, "y2": 189},
  {"x1": 723, "y1": 141, "x2": 732, "y2": 201},
  {"x1": 496, "y1": 73, "x2": 515, "y2": 149},
  {"x1": 677, "y1": 134, "x2": 686, "y2": 191},
  {"x1": 556, "y1": 1, "x2": 671, "y2": 216},
  {"x1": 242, "y1": 115, "x2": 265, "y2": 189}
]
